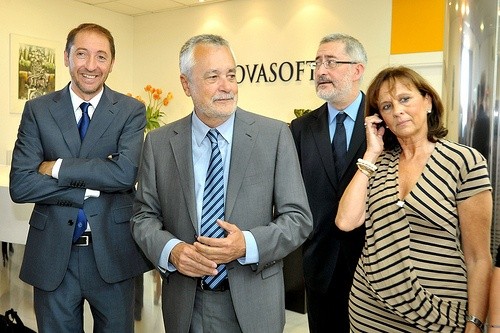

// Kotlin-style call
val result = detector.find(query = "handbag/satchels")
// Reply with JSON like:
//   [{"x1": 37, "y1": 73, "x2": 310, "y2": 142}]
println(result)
[{"x1": 0, "y1": 309, "x2": 37, "y2": 333}]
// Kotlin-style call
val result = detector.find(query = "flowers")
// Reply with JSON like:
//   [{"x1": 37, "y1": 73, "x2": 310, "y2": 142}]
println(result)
[{"x1": 127, "y1": 84, "x2": 174, "y2": 134}]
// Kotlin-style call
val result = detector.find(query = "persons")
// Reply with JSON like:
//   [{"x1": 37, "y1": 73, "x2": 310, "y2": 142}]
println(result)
[
  {"x1": 8, "y1": 23, "x2": 157, "y2": 333},
  {"x1": 335, "y1": 65, "x2": 491, "y2": 333},
  {"x1": 487, "y1": 242, "x2": 500, "y2": 333},
  {"x1": 289, "y1": 33, "x2": 368, "y2": 333},
  {"x1": 130, "y1": 34, "x2": 313, "y2": 333},
  {"x1": 463, "y1": 70, "x2": 500, "y2": 260}
]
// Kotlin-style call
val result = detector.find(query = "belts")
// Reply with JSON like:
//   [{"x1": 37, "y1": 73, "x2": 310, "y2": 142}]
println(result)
[
  {"x1": 197, "y1": 277, "x2": 229, "y2": 291},
  {"x1": 70, "y1": 231, "x2": 92, "y2": 246}
]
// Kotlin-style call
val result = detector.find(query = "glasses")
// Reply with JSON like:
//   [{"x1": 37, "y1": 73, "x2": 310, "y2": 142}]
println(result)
[{"x1": 309, "y1": 59, "x2": 357, "y2": 70}]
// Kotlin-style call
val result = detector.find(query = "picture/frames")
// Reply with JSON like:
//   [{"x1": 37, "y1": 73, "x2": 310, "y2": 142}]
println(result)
[{"x1": 9, "y1": 31, "x2": 61, "y2": 118}]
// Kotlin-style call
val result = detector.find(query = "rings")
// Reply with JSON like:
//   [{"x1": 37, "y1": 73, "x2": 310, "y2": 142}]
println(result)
[{"x1": 364, "y1": 123, "x2": 367, "y2": 129}]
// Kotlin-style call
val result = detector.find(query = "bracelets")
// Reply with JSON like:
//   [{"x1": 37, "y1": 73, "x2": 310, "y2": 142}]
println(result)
[
  {"x1": 356, "y1": 159, "x2": 377, "y2": 177},
  {"x1": 486, "y1": 324, "x2": 500, "y2": 328},
  {"x1": 466, "y1": 315, "x2": 485, "y2": 333}
]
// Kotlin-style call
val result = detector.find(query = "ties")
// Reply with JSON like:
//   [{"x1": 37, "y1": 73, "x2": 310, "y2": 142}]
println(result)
[
  {"x1": 331, "y1": 114, "x2": 348, "y2": 173},
  {"x1": 199, "y1": 128, "x2": 228, "y2": 291},
  {"x1": 70, "y1": 102, "x2": 93, "y2": 243}
]
